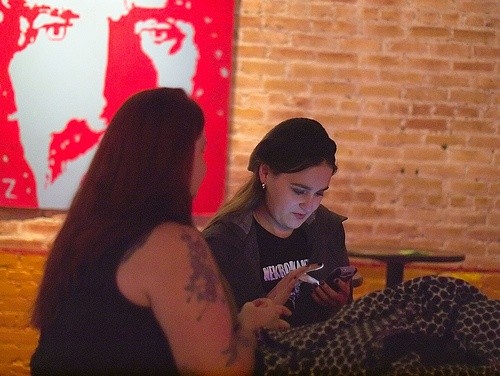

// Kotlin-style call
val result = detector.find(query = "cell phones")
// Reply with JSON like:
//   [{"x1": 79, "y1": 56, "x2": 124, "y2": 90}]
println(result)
[{"x1": 321, "y1": 267, "x2": 357, "y2": 292}]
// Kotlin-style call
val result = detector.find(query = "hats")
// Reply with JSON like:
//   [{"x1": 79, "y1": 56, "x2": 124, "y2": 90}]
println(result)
[{"x1": 248, "y1": 118, "x2": 337, "y2": 172}]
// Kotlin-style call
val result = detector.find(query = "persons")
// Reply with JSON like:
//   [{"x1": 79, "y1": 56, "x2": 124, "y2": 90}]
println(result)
[
  {"x1": 201, "y1": 118, "x2": 352, "y2": 334},
  {"x1": 29, "y1": 87, "x2": 291, "y2": 376}
]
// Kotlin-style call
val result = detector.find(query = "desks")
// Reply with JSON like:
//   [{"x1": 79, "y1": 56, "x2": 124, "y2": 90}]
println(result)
[{"x1": 344, "y1": 246, "x2": 466, "y2": 291}]
